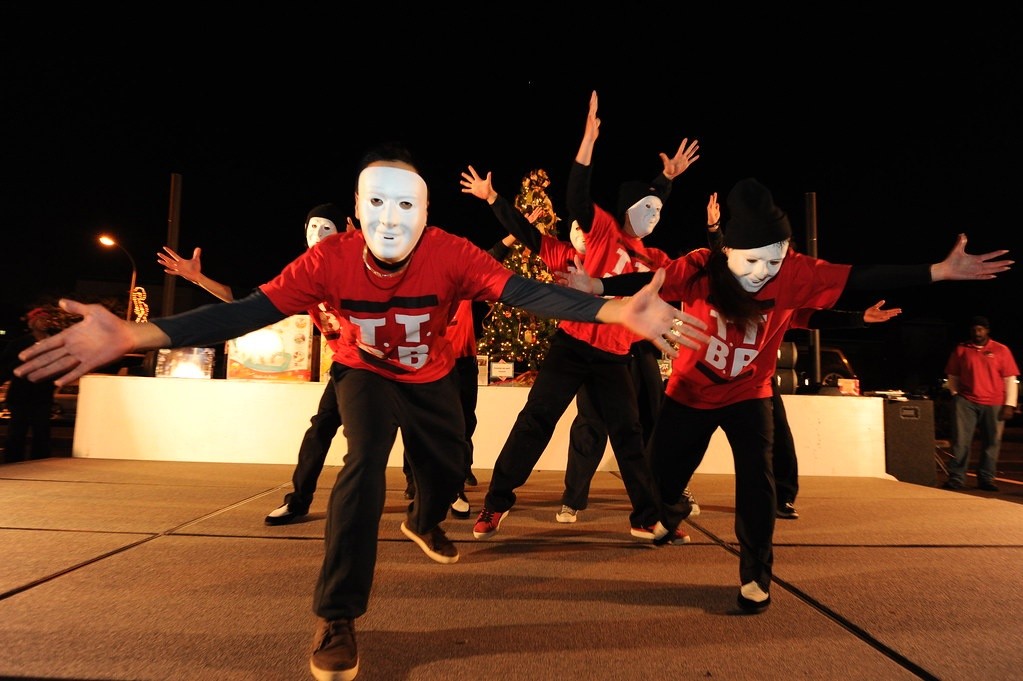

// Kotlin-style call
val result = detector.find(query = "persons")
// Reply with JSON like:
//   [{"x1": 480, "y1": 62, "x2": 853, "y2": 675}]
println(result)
[
  {"x1": 553, "y1": 179, "x2": 1016, "y2": 614},
  {"x1": 402, "y1": 206, "x2": 543, "y2": 499},
  {"x1": 707, "y1": 192, "x2": 902, "y2": 519},
  {"x1": 459, "y1": 137, "x2": 701, "y2": 522},
  {"x1": 473, "y1": 90, "x2": 701, "y2": 545},
  {"x1": 156, "y1": 202, "x2": 471, "y2": 525},
  {"x1": 14, "y1": 146, "x2": 713, "y2": 681},
  {"x1": 943, "y1": 316, "x2": 1020, "y2": 491},
  {"x1": 0, "y1": 312, "x2": 62, "y2": 464}
]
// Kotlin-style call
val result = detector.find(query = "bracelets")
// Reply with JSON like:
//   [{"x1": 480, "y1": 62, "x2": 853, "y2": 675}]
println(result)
[{"x1": 707, "y1": 221, "x2": 720, "y2": 228}]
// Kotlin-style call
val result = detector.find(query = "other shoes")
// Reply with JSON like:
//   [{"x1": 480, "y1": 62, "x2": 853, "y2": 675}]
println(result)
[
  {"x1": 465, "y1": 473, "x2": 477, "y2": 485},
  {"x1": 943, "y1": 479, "x2": 961, "y2": 490},
  {"x1": 404, "y1": 484, "x2": 416, "y2": 499},
  {"x1": 452, "y1": 492, "x2": 471, "y2": 518},
  {"x1": 977, "y1": 482, "x2": 999, "y2": 491},
  {"x1": 265, "y1": 503, "x2": 308, "y2": 524}
]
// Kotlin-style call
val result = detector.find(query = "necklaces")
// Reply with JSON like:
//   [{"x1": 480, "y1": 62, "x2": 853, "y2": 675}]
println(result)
[{"x1": 363, "y1": 245, "x2": 413, "y2": 279}]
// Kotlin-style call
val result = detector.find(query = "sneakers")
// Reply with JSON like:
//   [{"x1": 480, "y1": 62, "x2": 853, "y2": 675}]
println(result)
[
  {"x1": 556, "y1": 504, "x2": 579, "y2": 522},
  {"x1": 777, "y1": 502, "x2": 799, "y2": 518},
  {"x1": 400, "y1": 519, "x2": 459, "y2": 563},
  {"x1": 473, "y1": 506, "x2": 510, "y2": 538},
  {"x1": 681, "y1": 486, "x2": 700, "y2": 517},
  {"x1": 652, "y1": 497, "x2": 693, "y2": 546},
  {"x1": 310, "y1": 616, "x2": 359, "y2": 681},
  {"x1": 735, "y1": 576, "x2": 771, "y2": 612},
  {"x1": 631, "y1": 527, "x2": 690, "y2": 544}
]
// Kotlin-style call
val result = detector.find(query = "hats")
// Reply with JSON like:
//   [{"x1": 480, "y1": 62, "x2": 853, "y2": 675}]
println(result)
[{"x1": 972, "y1": 315, "x2": 990, "y2": 329}]
